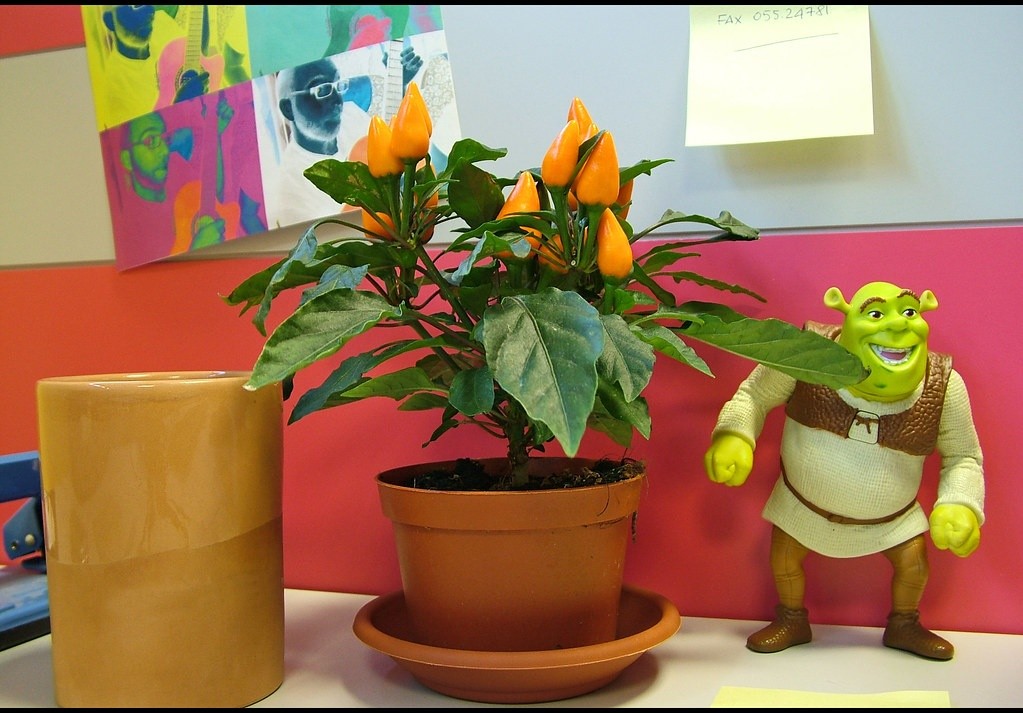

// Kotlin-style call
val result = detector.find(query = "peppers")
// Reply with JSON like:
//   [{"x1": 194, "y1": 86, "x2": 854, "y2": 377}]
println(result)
[{"x1": 361, "y1": 81, "x2": 638, "y2": 286}]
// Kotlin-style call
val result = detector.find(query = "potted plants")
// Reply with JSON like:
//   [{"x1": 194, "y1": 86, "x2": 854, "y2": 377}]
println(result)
[{"x1": 225, "y1": 82, "x2": 871, "y2": 653}]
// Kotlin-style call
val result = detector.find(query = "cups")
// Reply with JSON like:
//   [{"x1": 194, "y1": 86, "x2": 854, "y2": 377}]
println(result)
[{"x1": 34, "y1": 370, "x2": 286, "y2": 708}]
